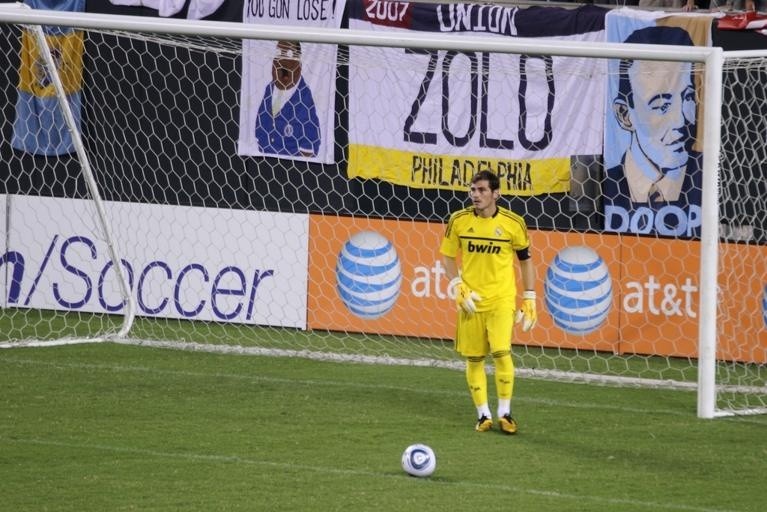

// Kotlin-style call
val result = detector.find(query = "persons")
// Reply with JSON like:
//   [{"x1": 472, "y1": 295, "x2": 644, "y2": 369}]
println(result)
[
  {"x1": 572, "y1": 0, "x2": 767, "y2": 14},
  {"x1": 438, "y1": 170, "x2": 538, "y2": 434},
  {"x1": 603, "y1": 25, "x2": 702, "y2": 236}
]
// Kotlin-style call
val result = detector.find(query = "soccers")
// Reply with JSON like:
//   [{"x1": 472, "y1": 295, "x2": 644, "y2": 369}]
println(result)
[{"x1": 401, "y1": 444, "x2": 436, "y2": 478}]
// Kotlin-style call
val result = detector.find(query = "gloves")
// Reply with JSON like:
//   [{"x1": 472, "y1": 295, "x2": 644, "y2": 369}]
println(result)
[
  {"x1": 516, "y1": 291, "x2": 536, "y2": 332},
  {"x1": 450, "y1": 277, "x2": 481, "y2": 313}
]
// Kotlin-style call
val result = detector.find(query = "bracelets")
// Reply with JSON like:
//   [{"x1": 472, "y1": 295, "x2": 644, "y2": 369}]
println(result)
[
  {"x1": 522, "y1": 289, "x2": 536, "y2": 299},
  {"x1": 450, "y1": 276, "x2": 463, "y2": 287}
]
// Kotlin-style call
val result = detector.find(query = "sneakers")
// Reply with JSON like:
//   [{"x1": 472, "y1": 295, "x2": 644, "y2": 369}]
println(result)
[
  {"x1": 499, "y1": 413, "x2": 517, "y2": 433},
  {"x1": 475, "y1": 415, "x2": 493, "y2": 432}
]
